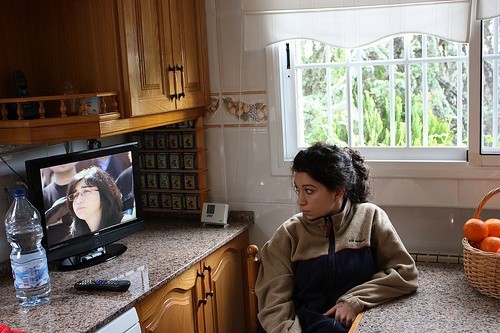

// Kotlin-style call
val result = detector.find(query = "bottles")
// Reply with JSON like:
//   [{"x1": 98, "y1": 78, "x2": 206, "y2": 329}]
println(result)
[{"x1": 4, "y1": 188, "x2": 52, "y2": 307}]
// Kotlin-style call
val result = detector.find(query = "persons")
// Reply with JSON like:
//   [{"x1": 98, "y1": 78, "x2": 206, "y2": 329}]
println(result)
[
  {"x1": 255, "y1": 141, "x2": 419, "y2": 333},
  {"x1": 40, "y1": 151, "x2": 136, "y2": 245}
]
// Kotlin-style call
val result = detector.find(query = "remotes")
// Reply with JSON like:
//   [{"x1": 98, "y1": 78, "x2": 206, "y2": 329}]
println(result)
[{"x1": 73, "y1": 279, "x2": 131, "y2": 293}]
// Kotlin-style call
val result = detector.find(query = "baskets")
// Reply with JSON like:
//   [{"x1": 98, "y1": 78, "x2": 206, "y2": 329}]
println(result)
[{"x1": 462, "y1": 186, "x2": 500, "y2": 299}]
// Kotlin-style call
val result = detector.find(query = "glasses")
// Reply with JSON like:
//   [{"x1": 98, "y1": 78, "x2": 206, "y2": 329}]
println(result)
[{"x1": 67, "y1": 188, "x2": 99, "y2": 201}]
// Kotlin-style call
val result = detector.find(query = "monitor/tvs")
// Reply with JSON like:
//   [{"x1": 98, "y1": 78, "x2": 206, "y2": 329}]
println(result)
[{"x1": 24, "y1": 141, "x2": 144, "y2": 269}]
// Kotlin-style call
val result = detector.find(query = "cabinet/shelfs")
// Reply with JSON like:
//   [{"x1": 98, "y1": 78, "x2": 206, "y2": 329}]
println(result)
[
  {"x1": 0, "y1": 0, "x2": 212, "y2": 119},
  {"x1": 135, "y1": 230, "x2": 250, "y2": 333}
]
